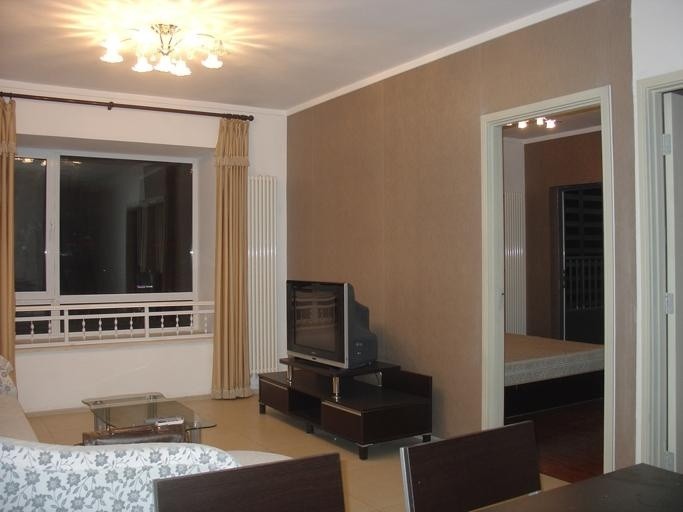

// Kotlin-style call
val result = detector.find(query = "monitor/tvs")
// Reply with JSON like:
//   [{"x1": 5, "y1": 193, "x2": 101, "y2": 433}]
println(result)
[{"x1": 286, "y1": 279, "x2": 377, "y2": 369}]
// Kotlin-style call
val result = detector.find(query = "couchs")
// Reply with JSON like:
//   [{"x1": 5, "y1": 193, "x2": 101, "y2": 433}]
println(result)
[{"x1": 0, "y1": 352, "x2": 296, "y2": 511}]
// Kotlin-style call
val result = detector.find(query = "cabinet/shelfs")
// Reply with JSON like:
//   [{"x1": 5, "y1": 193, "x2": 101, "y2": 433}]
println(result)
[{"x1": 251, "y1": 358, "x2": 434, "y2": 461}]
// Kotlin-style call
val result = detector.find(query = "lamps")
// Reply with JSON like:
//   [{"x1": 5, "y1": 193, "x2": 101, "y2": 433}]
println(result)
[
  {"x1": 507, "y1": 115, "x2": 559, "y2": 135},
  {"x1": 95, "y1": 24, "x2": 230, "y2": 79}
]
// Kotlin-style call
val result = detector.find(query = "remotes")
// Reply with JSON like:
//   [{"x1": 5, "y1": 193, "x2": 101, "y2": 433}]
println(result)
[{"x1": 156, "y1": 416, "x2": 184, "y2": 426}]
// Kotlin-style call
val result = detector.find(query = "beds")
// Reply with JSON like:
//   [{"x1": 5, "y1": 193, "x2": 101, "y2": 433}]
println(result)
[{"x1": 504, "y1": 326, "x2": 604, "y2": 416}]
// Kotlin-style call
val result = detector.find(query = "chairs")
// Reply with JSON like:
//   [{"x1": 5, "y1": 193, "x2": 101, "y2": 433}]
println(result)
[
  {"x1": 151, "y1": 451, "x2": 347, "y2": 512},
  {"x1": 398, "y1": 417, "x2": 545, "y2": 512}
]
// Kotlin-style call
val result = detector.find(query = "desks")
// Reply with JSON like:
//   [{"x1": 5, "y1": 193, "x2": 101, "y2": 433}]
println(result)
[{"x1": 467, "y1": 458, "x2": 682, "y2": 512}]
